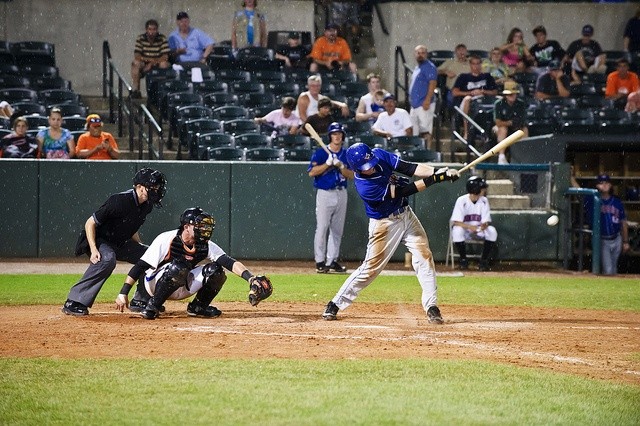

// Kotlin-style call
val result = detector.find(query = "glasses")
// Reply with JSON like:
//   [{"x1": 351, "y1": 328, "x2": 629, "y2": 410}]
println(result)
[{"x1": 88, "y1": 118, "x2": 102, "y2": 123}]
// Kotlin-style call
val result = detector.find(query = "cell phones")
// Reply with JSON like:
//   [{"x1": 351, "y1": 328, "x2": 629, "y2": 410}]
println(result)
[{"x1": 102, "y1": 137, "x2": 112, "y2": 143}]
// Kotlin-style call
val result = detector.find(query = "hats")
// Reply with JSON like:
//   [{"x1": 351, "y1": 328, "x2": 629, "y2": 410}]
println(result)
[
  {"x1": 502, "y1": 81, "x2": 520, "y2": 93},
  {"x1": 548, "y1": 59, "x2": 561, "y2": 69},
  {"x1": 318, "y1": 98, "x2": 331, "y2": 108},
  {"x1": 289, "y1": 30, "x2": 300, "y2": 39},
  {"x1": 86, "y1": 113, "x2": 101, "y2": 126},
  {"x1": 383, "y1": 93, "x2": 396, "y2": 101},
  {"x1": 326, "y1": 23, "x2": 337, "y2": 29},
  {"x1": 177, "y1": 11, "x2": 188, "y2": 19},
  {"x1": 581, "y1": 23, "x2": 594, "y2": 36},
  {"x1": 283, "y1": 97, "x2": 295, "y2": 108},
  {"x1": 596, "y1": 174, "x2": 612, "y2": 184}
]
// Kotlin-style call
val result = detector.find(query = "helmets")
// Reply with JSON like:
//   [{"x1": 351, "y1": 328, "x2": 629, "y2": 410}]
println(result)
[
  {"x1": 177, "y1": 205, "x2": 215, "y2": 251},
  {"x1": 466, "y1": 175, "x2": 489, "y2": 195},
  {"x1": 327, "y1": 121, "x2": 346, "y2": 142},
  {"x1": 346, "y1": 142, "x2": 380, "y2": 171},
  {"x1": 131, "y1": 168, "x2": 167, "y2": 209}
]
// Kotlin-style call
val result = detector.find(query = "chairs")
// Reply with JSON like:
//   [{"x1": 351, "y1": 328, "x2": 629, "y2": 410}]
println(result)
[
  {"x1": 388, "y1": 136, "x2": 427, "y2": 152},
  {"x1": 427, "y1": 49, "x2": 454, "y2": 87},
  {"x1": 468, "y1": 49, "x2": 488, "y2": 58},
  {"x1": 401, "y1": 150, "x2": 443, "y2": 163},
  {"x1": 145, "y1": 40, "x2": 387, "y2": 160},
  {"x1": 0, "y1": 40, "x2": 89, "y2": 147},
  {"x1": 469, "y1": 75, "x2": 640, "y2": 171},
  {"x1": 445, "y1": 222, "x2": 484, "y2": 269}
]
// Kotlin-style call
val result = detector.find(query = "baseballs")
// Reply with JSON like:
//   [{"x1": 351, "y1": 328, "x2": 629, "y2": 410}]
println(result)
[{"x1": 547, "y1": 214, "x2": 559, "y2": 227}]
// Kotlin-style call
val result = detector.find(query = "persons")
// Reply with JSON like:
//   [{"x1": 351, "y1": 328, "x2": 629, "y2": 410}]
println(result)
[
  {"x1": 449, "y1": 175, "x2": 498, "y2": 271},
  {"x1": 500, "y1": 27, "x2": 530, "y2": 73},
  {"x1": 0, "y1": 117, "x2": 40, "y2": 158},
  {"x1": 372, "y1": 93, "x2": 412, "y2": 138},
  {"x1": 571, "y1": 173, "x2": 630, "y2": 275},
  {"x1": 307, "y1": 121, "x2": 355, "y2": 273},
  {"x1": 438, "y1": 43, "x2": 472, "y2": 106},
  {"x1": 492, "y1": 81, "x2": 529, "y2": 165},
  {"x1": 534, "y1": 60, "x2": 571, "y2": 98},
  {"x1": 309, "y1": 23, "x2": 357, "y2": 73},
  {"x1": 76, "y1": 114, "x2": 120, "y2": 160},
  {"x1": 323, "y1": 142, "x2": 460, "y2": 323},
  {"x1": 231, "y1": 0, "x2": 267, "y2": 50},
  {"x1": 605, "y1": 58, "x2": 640, "y2": 110},
  {"x1": 37, "y1": 108, "x2": 75, "y2": 158},
  {"x1": 275, "y1": 31, "x2": 309, "y2": 68},
  {"x1": 623, "y1": 8, "x2": 640, "y2": 63},
  {"x1": 61, "y1": 167, "x2": 166, "y2": 316},
  {"x1": 168, "y1": 12, "x2": 216, "y2": 64},
  {"x1": 254, "y1": 97, "x2": 300, "y2": 135},
  {"x1": 296, "y1": 75, "x2": 348, "y2": 124},
  {"x1": 115, "y1": 206, "x2": 273, "y2": 319},
  {"x1": 484, "y1": 46, "x2": 508, "y2": 84},
  {"x1": 408, "y1": 45, "x2": 438, "y2": 150},
  {"x1": 452, "y1": 54, "x2": 499, "y2": 145},
  {"x1": 301, "y1": 98, "x2": 335, "y2": 135},
  {"x1": 131, "y1": 19, "x2": 173, "y2": 97},
  {"x1": 356, "y1": 72, "x2": 391, "y2": 121},
  {"x1": 529, "y1": 25, "x2": 566, "y2": 67},
  {"x1": 559, "y1": 24, "x2": 607, "y2": 84},
  {"x1": 370, "y1": 91, "x2": 385, "y2": 122}
]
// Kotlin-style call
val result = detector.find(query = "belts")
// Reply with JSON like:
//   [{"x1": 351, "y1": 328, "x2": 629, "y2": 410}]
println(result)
[
  {"x1": 330, "y1": 186, "x2": 347, "y2": 190},
  {"x1": 385, "y1": 206, "x2": 406, "y2": 218}
]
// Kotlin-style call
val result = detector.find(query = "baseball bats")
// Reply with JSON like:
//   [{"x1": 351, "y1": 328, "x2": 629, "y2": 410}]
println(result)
[
  {"x1": 450, "y1": 129, "x2": 525, "y2": 184},
  {"x1": 304, "y1": 122, "x2": 332, "y2": 155}
]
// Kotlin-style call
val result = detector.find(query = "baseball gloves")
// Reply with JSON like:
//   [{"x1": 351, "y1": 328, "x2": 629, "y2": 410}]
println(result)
[{"x1": 248, "y1": 275, "x2": 273, "y2": 307}]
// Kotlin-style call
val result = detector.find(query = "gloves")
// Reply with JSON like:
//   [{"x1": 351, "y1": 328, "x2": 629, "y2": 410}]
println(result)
[
  {"x1": 423, "y1": 170, "x2": 461, "y2": 187},
  {"x1": 335, "y1": 159, "x2": 345, "y2": 169},
  {"x1": 326, "y1": 152, "x2": 338, "y2": 166},
  {"x1": 434, "y1": 167, "x2": 459, "y2": 182}
]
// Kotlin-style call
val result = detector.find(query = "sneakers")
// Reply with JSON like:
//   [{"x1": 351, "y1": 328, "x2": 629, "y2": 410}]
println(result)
[
  {"x1": 61, "y1": 298, "x2": 90, "y2": 316},
  {"x1": 315, "y1": 261, "x2": 329, "y2": 272},
  {"x1": 478, "y1": 265, "x2": 491, "y2": 271},
  {"x1": 323, "y1": 301, "x2": 339, "y2": 321},
  {"x1": 129, "y1": 298, "x2": 166, "y2": 313},
  {"x1": 498, "y1": 157, "x2": 508, "y2": 165},
  {"x1": 186, "y1": 300, "x2": 222, "y2": 319},
  {"x1": 139, "y1": 298, "x2": 159, "y2": 319},
  {"x1": 327, "y1": 258, "x2": 347, "y2": 271},
  {"x1": 426, "y1": 306, "x2": 445, "y2": 324},
  {"x1": 454, "y1": 263, "x2": 470, "y2": 270}
]
